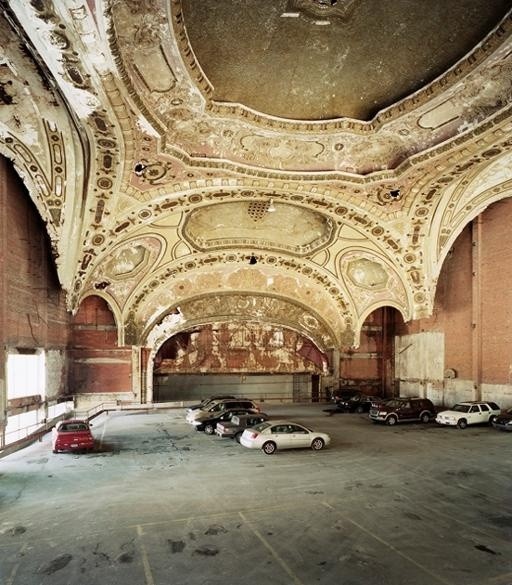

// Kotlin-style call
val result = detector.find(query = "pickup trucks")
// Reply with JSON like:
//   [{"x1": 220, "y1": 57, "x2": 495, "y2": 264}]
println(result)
[{"x1": 434, "y1": 401, "x2": 501, "y2": 430}]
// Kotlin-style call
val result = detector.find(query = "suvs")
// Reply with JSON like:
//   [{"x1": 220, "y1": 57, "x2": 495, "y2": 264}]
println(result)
[
  {"x1": 185, "y1": 394, "x2": 235, "y2": 417},
  {"x1": 186, "y1": 398, "x2": 260, "y2": 425},
  {"x1": 369, "y1": 397, "x2": 435, "y2": 426},
  {"x1": 330, "y1": 388, "x2": 366, "y2": 405}
]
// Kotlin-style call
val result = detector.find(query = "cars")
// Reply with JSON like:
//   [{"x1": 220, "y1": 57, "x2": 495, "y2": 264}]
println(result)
[
  {"x1": 214, "y1": 414, "x2": 270, "y2": 444},
  {"x1": 338, "y1": 394, "x2": 384, "y2": 412},
  {"x1": 52, "y1": 419, "x2": 96, "y2": 455},
  {"x1": 491, "y1": 407, "x2": 512, "y2": 433},
  {"x1": 193, "y1": 408, "x2": 257, "y2": 435},
  {"x1": 370, "y1": 397, "x2": 399, "y2": 408},
  {"x1": 239, "y1": 420, "x2": 330, "y2": 455}
]
